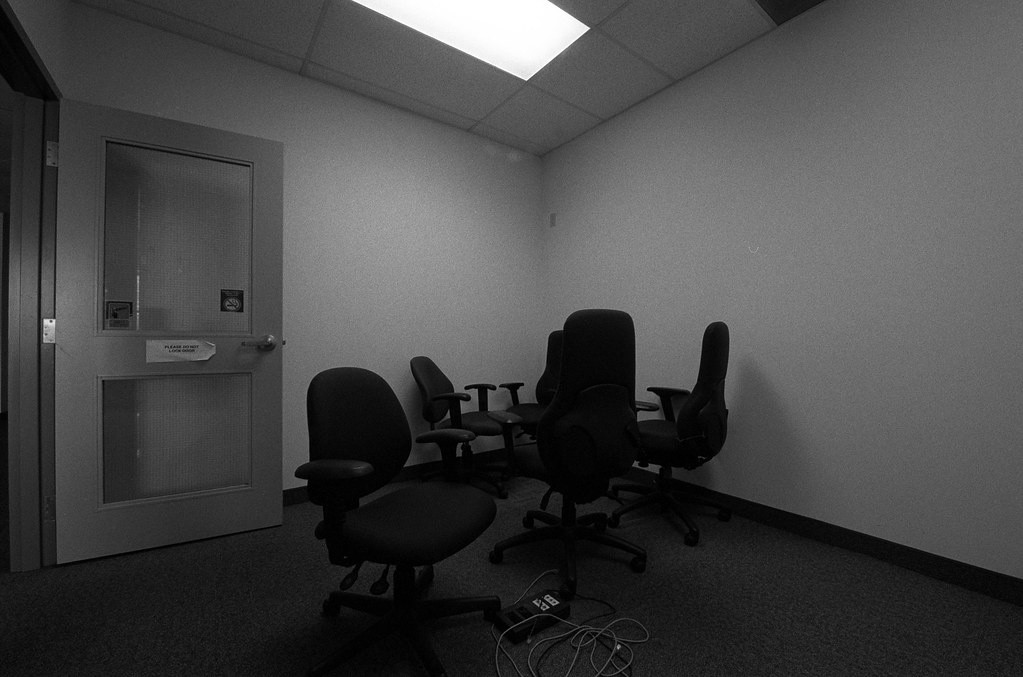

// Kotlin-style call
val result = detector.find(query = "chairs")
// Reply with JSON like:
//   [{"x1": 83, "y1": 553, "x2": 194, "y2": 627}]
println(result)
[
  {"x1": 500, "y1": 329, "x2": 565, "y2": 460},
  {"x1": 409, "y1": 356, "x2": 524, "y2": 499},
  {"x1": 294, "y1": 367, "x2": 502, "y2": 677},
  {"x1": 489, "y1": 309, "x2": 649, "y2": 603},
  {"x1": 603, "y1": 322, "x2": 731, "y2": 547}
]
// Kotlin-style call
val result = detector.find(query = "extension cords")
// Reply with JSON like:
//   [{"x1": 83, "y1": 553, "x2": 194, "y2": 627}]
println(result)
[{"x1": 496, "y1": 590, "x2": 569, "y2": 643}]
